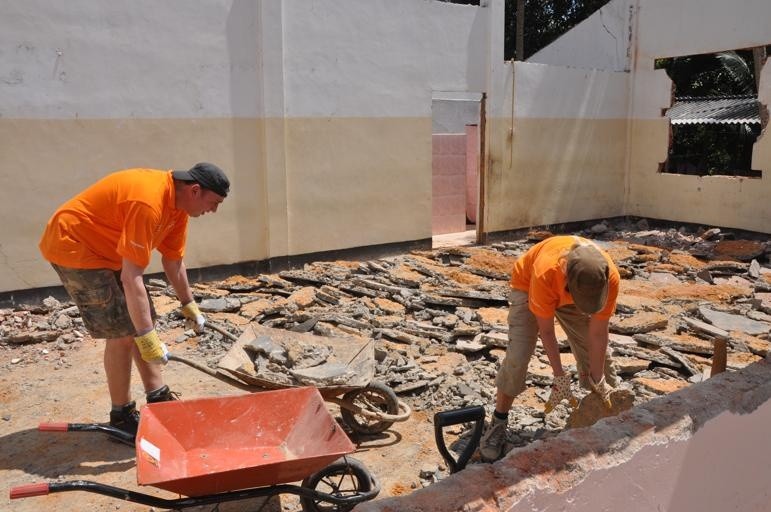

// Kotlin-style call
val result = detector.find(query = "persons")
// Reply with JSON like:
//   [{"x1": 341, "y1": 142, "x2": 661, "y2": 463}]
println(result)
[
  {"x1": 478, "y1": 234, "x2": 620, "y2": 464},
  {"x1": 38, "y1": 161, "x2": 230, "y2": 445}
]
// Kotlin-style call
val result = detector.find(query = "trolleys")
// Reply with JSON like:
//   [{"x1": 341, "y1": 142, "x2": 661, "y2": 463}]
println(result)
[
  {"x1": 167, "y1": 314, "x2": 413, "y2": 436},
  {"x1": 8, "y1": 385, "x2": 379, "y2": 512}
]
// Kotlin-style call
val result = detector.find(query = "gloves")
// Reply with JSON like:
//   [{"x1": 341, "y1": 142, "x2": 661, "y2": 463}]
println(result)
[
  {"x1": 588, "y1": 371, "x2": 618, "y2": 409},
  {"x1": 544, "y1": 373, "x2": 579, "y2": 415},
  {"x1": 133, "y1": 327, "x2": 173, "y2": 365},
  {"x1": 180, "y1": 299, "x2": 206, "y2": 336}
]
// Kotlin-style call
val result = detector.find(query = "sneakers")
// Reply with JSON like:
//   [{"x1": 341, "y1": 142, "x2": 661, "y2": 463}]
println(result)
[
  {"x1": 478, "y1": 412, "x2": 508, "y2": 463},
  {"x1": 108, "y1": 400, "x2": 142, "y2": 445},
  {"x1": 143, "y1": 384, "x2": 179, "y2": 405}
]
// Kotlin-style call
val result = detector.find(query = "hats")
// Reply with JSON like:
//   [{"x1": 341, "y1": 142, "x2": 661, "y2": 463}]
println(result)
[
  {"x1": 565, "y1": 245, "x2": 611, "y2": 316},
  {"x1": 172, "y1": 162, "x2": 231, "y2": 198}
]
